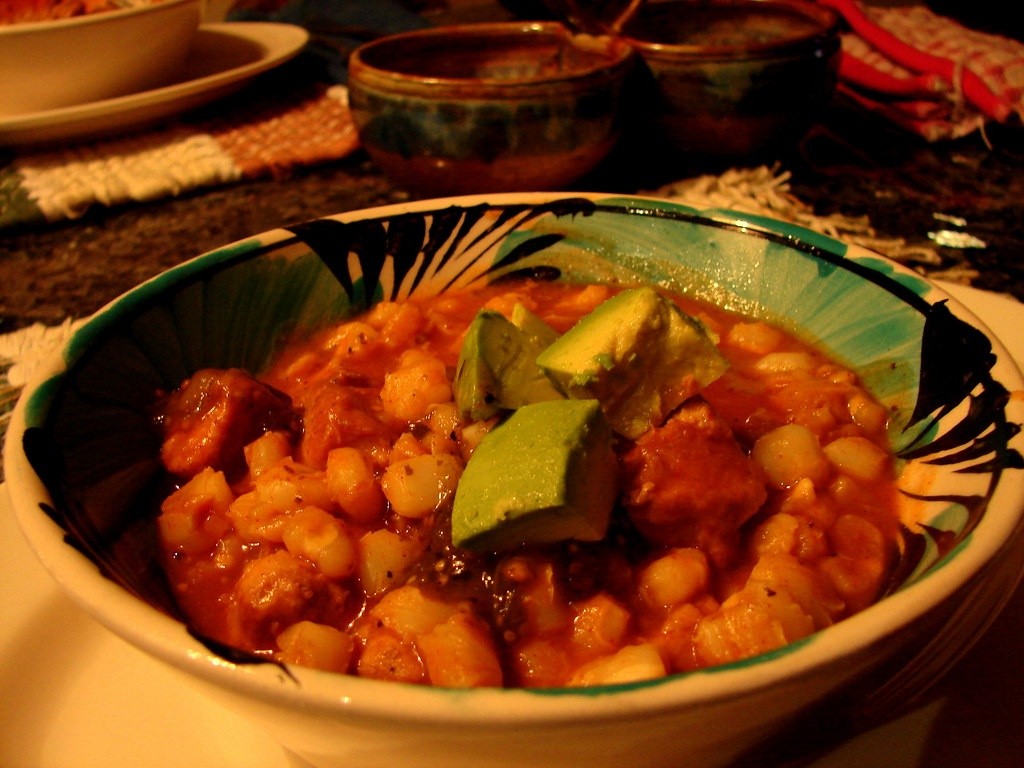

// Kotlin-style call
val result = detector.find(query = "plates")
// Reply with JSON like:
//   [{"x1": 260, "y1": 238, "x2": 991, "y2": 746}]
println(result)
[{"x1": 0, "y1": 21, "x2": 311, "y2": 149}]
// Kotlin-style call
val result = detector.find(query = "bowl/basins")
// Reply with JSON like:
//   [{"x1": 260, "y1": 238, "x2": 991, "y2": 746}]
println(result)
[
  {"x1": 349, "y1": 21, "x2": 636, "y2": 198},
  {"x1": 567, "y1": 0, "x2": 841, "y2": 166},
  {"x1": 0, "y1": 0, "x2": 198, "y2": 116},
  {"x1": 5, "y1": 192, "x2": 1023, "y2": 768}
]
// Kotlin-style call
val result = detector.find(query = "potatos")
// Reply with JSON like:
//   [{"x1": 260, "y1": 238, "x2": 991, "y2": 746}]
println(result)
[
  {"x1": 576, "y1": 284, "x2": 610, "y2": 306},
  {"x1": 160, "y1": 287, "x2": 535, "y2": 696},
  {"x1": 512, "y1": 320, "x2": 897, "y2": 685}
]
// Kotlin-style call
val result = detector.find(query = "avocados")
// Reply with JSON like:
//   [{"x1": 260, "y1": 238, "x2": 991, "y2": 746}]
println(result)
[{"x1": 451, "y1": 284, "x2": 728, "y2": 551}]
